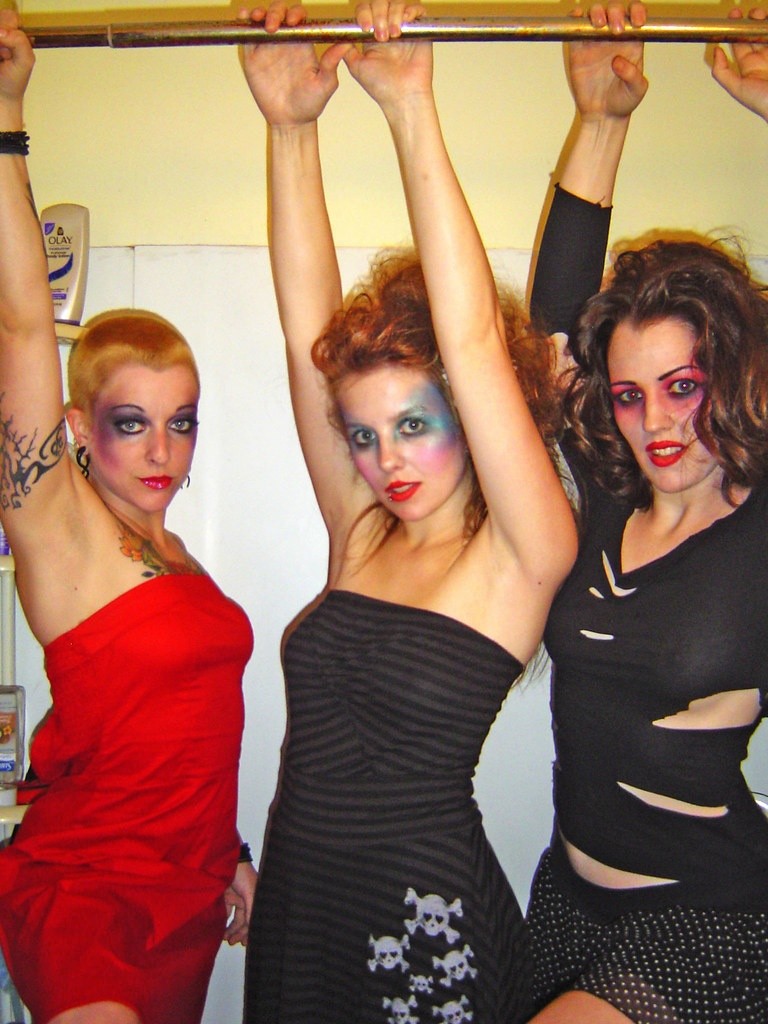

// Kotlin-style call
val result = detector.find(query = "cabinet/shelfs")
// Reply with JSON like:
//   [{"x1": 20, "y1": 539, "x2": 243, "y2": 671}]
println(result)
[{"x1": 1, "y1": 324, "x2": 88, "y2": 1024}]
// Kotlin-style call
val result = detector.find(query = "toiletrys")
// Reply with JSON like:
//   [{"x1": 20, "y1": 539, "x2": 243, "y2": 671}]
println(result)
[
  {"x1": 30, "y1": 202, "x2": 90, "y2": 327},
  {"x1": 0, "y1": 685, "x2": 26, "y2": 790}
]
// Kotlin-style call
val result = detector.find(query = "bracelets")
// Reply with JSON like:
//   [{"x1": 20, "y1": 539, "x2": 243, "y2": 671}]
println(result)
[
  {"x1": 0, "y1": 130, "x2": 30, "y2": 157},
  {"x1": 238, "y1": 842, "x2": 254, "y2": 863}
]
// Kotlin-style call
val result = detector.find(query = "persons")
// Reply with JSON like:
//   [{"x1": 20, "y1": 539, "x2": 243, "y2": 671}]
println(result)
[
  {"x1": 0, "y1": 0, "x2": 258, "y2": 1024},
  {"x1": 527, "y1": 1, "x2": 768, "y2": 1024},
  {"x1": 243, "y1": 2, "x2": 578, "y2": 1024}
]
